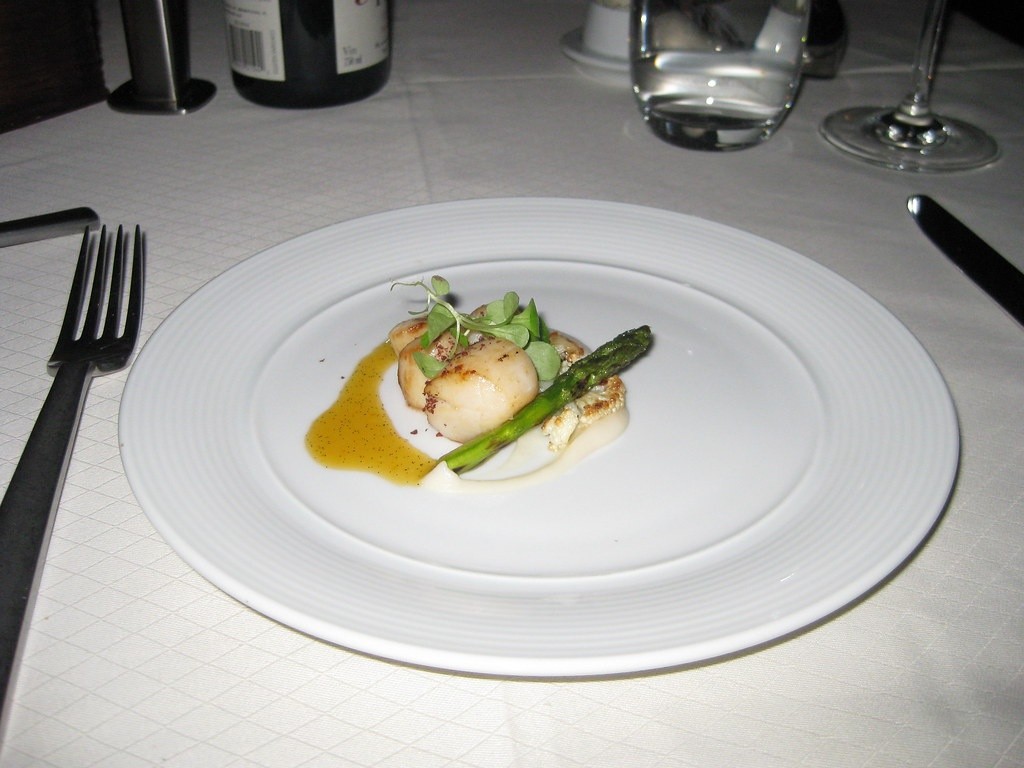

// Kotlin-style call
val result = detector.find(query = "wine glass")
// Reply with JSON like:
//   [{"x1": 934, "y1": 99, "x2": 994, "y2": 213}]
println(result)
[{"x1": 817, "y1": 0, "x2": 1004, "y2": 175}]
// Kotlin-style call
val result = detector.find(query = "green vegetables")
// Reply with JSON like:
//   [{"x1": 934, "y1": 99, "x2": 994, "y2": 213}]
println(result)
[{"x1": 389, "y1": 273, "x2": 560, "y2": 382}]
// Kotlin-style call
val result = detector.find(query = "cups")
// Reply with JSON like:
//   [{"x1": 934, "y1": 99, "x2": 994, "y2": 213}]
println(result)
[
  {"x1": 580, "y1": 0, "x2": 631, "y2": 61},
  {"x1": 627, "y1": 1, "x2": 812, "y2": 152}
]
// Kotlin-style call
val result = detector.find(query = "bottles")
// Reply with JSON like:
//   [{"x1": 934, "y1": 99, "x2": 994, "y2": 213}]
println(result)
[{"x1": 221, "y1": 0, "x2": 392, "y2": 111}]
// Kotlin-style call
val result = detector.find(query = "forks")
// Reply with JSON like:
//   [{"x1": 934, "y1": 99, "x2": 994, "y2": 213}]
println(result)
[{"x1": 0, "y1": 223, "x2": 146, "y2": 725}]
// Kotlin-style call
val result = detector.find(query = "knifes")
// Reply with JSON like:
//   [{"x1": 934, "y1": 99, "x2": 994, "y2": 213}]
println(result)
[{"x1": 905, "y1": 194, "x2": 1024, "y2": 329}]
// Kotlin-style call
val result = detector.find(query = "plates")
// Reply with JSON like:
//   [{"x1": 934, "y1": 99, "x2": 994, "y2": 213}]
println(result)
[
  {"x1": 558, "y1": 25, "x2": 630, "y2": 73},
  {"x1": 119, "y1": 197, "x2": 961, "y2": 680}
]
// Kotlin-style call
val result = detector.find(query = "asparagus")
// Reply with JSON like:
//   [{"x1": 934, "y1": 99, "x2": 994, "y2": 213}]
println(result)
[{"x1": 439, "y1": 325, "x2": 649, "y2": 476}]
